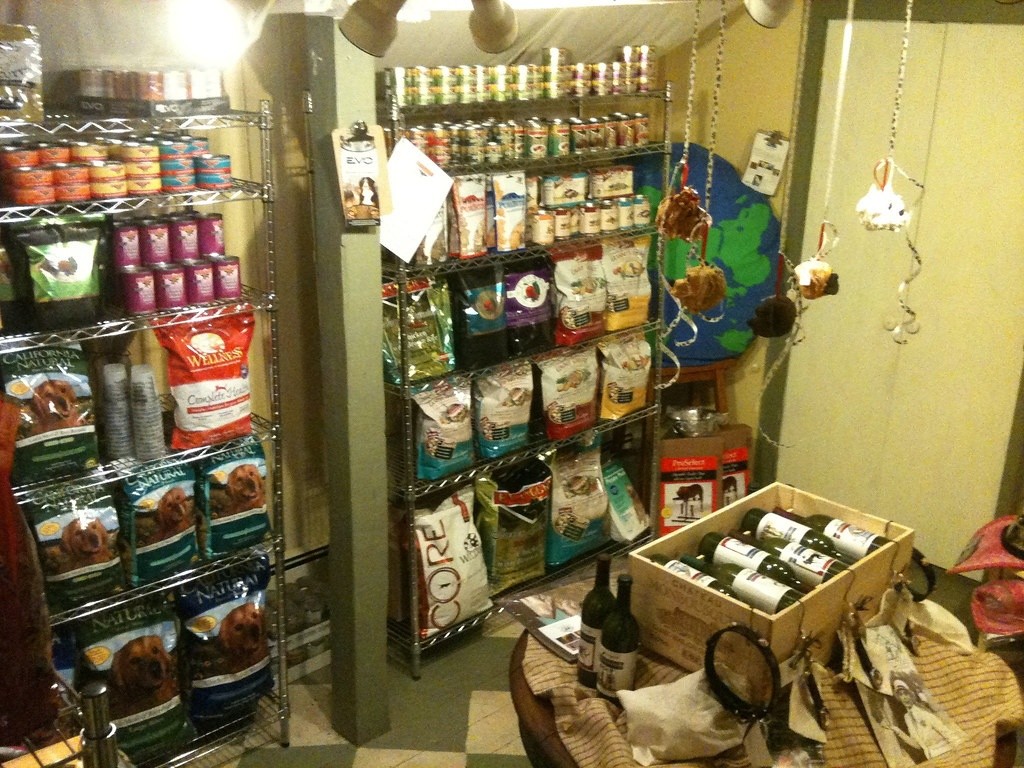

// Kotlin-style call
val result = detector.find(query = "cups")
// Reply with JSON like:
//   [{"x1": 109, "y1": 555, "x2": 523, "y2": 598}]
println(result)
[
  {"x1": 102, "y1": 363, "x2": 134, "y2": 460},
  {"x1": 128, "y1": 365, "x2": 166, "y2": 460}
]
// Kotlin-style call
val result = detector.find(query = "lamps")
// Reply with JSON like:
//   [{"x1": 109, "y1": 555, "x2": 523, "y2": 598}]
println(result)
[
  {"x1": 338, "y1": 0, "x2": 407, "y2": 58},
  {"x1": 744, "y1": 0, "x2": 792, "y2": 29},
  {"x1": 468, "y1": 0, "x2": 518, "y2": 54}
]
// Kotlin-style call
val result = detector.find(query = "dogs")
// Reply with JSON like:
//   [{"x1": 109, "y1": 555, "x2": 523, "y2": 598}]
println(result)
[
  {"x1": 150, "y1": 487, "x2": 191, "y2": 543},
  {"x1": 225, "y1": 463, "x2": 263, "y2": 513},
  {"x1": 112, "y1": 635, "x2": 176, "y2": 714},
  {"x1": 219, "y1": 601, "x2": 270, "y2": 675},
  {"x1": 25, "y1": 378, "x2": 82, "y2": 435},
  {"x1": 57, "y1": 515, "x2": 112, "y2": 574}
]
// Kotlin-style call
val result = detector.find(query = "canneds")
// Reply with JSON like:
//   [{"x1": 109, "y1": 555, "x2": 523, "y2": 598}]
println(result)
[
  {"x1": 114, "y1": 209, "x2": 242, "y2": 313},
  {"x1": 386, "y1": 44, "x2": 657, "y2": 166},
  {"x1": 0, "y1": 131, "x2": 233, "y2": 204},
  {"x1": 527, "y1": 168, "x2": 650, "y2": 246}
]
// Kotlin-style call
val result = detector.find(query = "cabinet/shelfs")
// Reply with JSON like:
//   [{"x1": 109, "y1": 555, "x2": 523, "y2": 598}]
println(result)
[
  {"x1": 0, "y1": 96, "x2": 291, "y2": 768},
  {"x1": 377, "y1": 80, "x2": 672, "y2": 681}
]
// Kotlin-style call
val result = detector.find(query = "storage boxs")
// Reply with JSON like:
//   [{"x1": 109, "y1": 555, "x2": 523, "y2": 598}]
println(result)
[
  {"x1": 71, "y1": 92, "x2": 231, "y2": 116},
  {"x1": 628, "y1": 480, "x2": 916, "y2": 711},
  {"x1": 658, "y1": 421, "x2": 754, "y2": 538}
]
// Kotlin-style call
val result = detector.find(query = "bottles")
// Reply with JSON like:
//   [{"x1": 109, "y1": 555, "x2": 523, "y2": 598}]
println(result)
[
  {"x1": 649, "y1": 552, "x2": 738, "y2": 605},
  {"x1": 577, "y1": 554, "x2": 616, "y2": 689},
  {"x1": 741, "y1": 509, "x2": 859, "y2": 565},
  {"x1": 772, "y1": 506, "x2": 890, "y2": 560},
  {"x1": 697, "y1": 532, "x2": 815, "y2": 594},
  {"x1": 727, "y1": 527, "x2": 848, "y2": 584},
  {"x1": 680, "y1": 555, "x2": 806, "y2": 615},
  {"x1": 595, "y1": 574, "x2": 640, "y2": 709}
]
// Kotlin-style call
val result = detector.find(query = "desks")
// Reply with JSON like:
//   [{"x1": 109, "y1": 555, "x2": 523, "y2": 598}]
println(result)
[
  {"x1": 611, "y1": 357, "x2": 738, "y2": 515},
  {"x1": 509, "y1": 558, "x2": 1024, "y2": 768}
]
